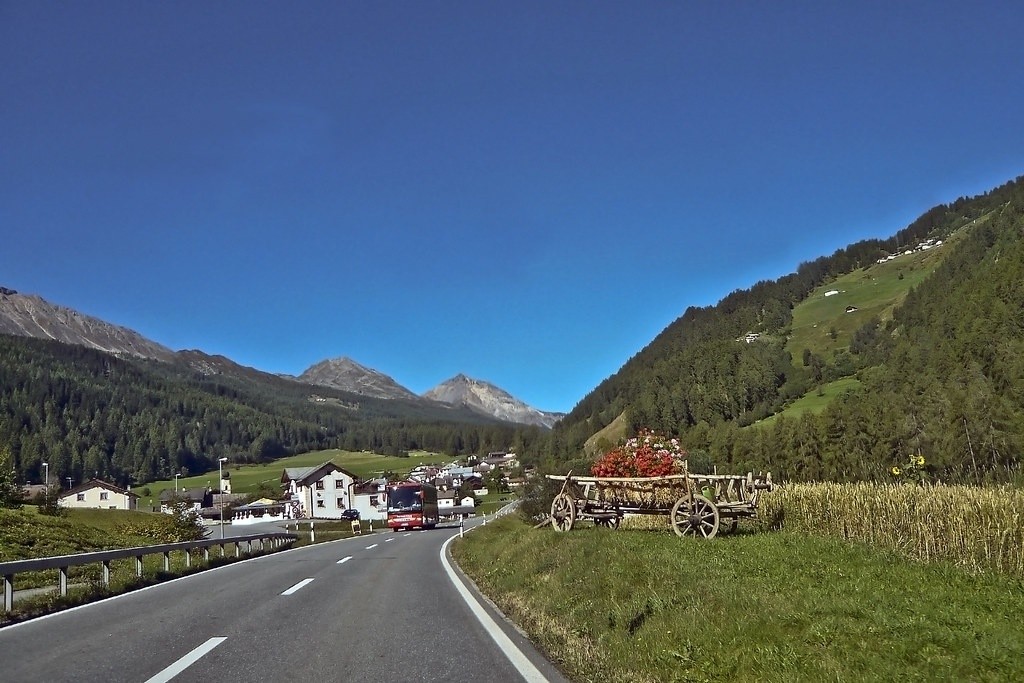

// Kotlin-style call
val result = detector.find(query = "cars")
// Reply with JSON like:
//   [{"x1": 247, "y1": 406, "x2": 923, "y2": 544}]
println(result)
[{"x1": 341, "y1": 509, "x2": 360, "y2": 520}]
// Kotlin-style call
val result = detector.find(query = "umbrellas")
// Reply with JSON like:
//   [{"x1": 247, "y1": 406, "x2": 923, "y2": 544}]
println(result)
[{"x1": 232, "y1": 498, "x2": 282, "y2": 515}]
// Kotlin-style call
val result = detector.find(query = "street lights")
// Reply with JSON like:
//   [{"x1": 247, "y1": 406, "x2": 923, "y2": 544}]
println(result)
[
  {"x1": 176, "y1": 473, "x2": 181, "y2": 497},
  {"x1": 41, "y1": 462, "x2": 48, "y2": 507},
  {"x1": 66, "y1": 477, "x2": 71, "y2": 490},
  {"x1": 219, "y1": 456, "x2": 227, "y2": 538}
]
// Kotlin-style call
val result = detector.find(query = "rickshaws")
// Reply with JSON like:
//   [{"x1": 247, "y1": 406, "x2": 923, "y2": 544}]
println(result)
[{"x1": 544, "y1": 459, "x2": 774, "y2": 539}]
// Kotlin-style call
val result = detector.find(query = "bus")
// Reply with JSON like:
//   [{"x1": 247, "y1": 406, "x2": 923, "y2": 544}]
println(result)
[{"x1": 385, "y1": 482, "x2": 439, "y2": 532}]
